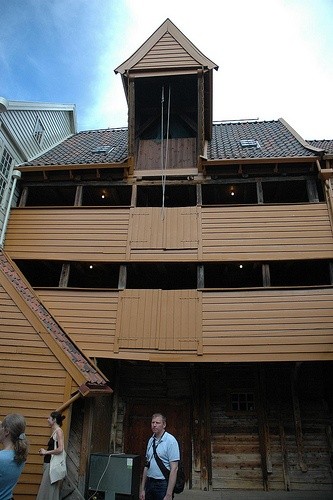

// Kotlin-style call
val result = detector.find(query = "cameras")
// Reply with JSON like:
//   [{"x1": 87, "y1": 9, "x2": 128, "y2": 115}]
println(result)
[{"x1": 144, "y1": 461, "x2": 150, "y2": 467}]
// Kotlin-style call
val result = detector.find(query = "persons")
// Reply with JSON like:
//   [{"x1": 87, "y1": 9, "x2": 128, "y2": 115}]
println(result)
[
  {"x1": 35, "y1": 411, "x2": 65, "y2": 500},
  {"x1": 139, "y1": 412, "x2": 180, "y2": 500},
  {"x1": 0, "y1": 413, "x2": 28, "y2": 500}
]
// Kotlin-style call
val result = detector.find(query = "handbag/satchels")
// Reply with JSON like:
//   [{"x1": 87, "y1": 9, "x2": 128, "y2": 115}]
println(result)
[
  {"x1": 49, "y1": 433, "x2": 67, "y2": 485},
  {"x1": 168, "y1": 469, "x2": 185, "y2": 494}
]
